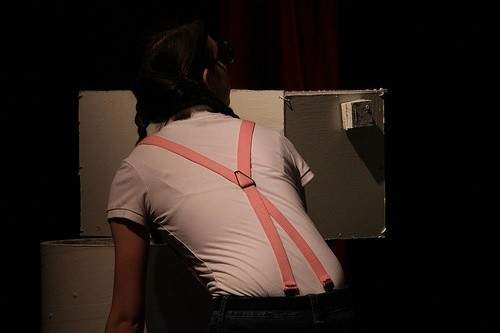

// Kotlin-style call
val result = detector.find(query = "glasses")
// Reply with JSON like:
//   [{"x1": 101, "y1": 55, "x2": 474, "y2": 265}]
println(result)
[{"x1": 210, "y1": 38, "x2": 236, "y2": 67}]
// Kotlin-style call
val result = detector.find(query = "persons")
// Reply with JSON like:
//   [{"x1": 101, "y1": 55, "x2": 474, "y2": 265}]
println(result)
[{"x1": 105, "y1": 17, "x2": 355, "y2": 333}]
